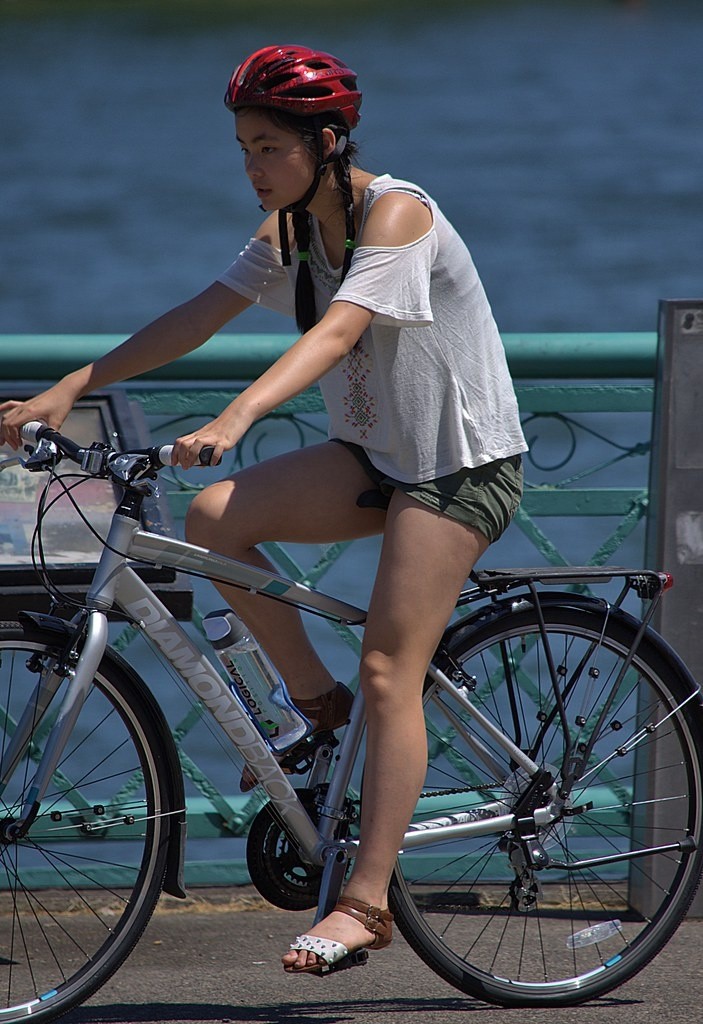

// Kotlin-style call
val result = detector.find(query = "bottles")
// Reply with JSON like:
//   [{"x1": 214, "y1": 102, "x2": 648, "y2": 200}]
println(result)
[{"x1": 201, "y1": 609, "x2": 307, "y2": 749}]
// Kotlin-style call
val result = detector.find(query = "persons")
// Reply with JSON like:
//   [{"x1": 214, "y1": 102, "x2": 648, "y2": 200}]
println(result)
[{"x1": 0, "y1": 46, "x2": 530, "y2": 973}]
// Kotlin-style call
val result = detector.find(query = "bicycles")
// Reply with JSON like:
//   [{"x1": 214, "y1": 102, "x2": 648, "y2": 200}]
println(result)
[{"x1": 0, "y1": 419, "x2": 702, "y2": 1024}]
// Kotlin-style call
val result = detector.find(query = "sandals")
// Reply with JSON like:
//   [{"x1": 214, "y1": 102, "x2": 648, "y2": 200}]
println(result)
[
  {"x1": 284, "y1": 896, "x2": 393, "y2": 973},
  {"x1": 240, "y1": 682, "x2": 354, "y2": 792}
]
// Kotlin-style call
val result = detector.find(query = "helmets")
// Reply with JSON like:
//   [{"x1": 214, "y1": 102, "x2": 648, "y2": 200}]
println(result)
[{"x1": 223, "y1": 45, "x2": 362, "y2": 129}]
